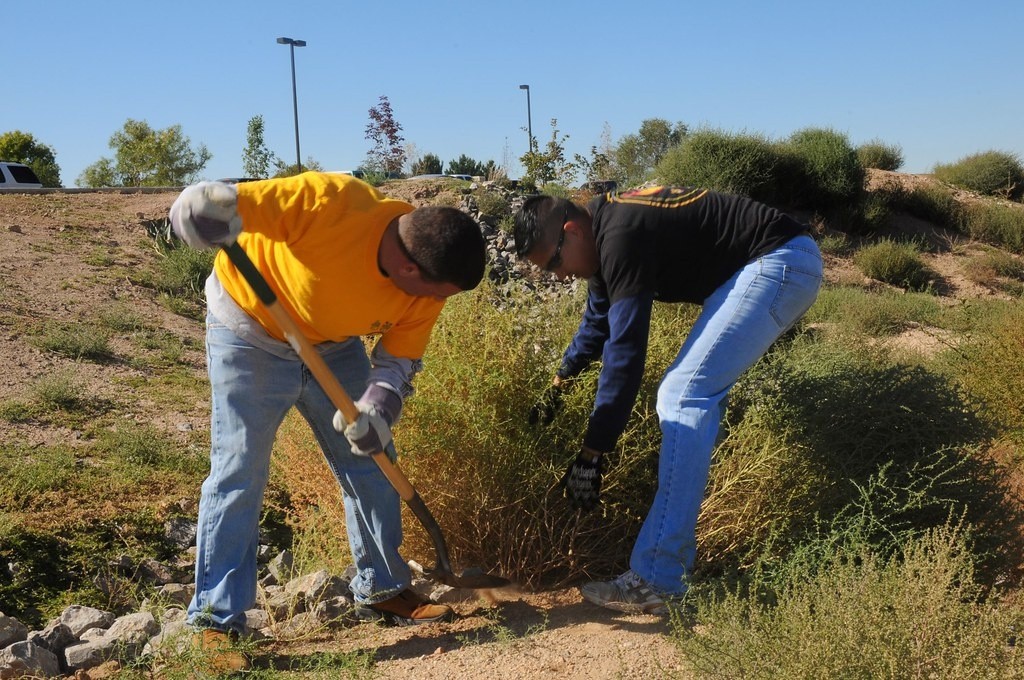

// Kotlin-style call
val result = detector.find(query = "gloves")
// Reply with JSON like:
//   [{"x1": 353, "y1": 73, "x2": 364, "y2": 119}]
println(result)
[
  {"x1": 171, "y1": 179, "x2": 243, "y2": 250},
  {"x1": 559, "y1": 450, "x2": 608, "y2": 514},
  {"x1": 527, "y1": 383, "x2": 565, "y2": 428},
  {"x1": 332, "y1": 382, "x2": 404, "y2": 457}
]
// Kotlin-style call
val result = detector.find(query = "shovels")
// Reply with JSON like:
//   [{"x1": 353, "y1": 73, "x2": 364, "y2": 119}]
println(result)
[{"x1": 225, "y1": 239, "x2": 513, "y2": 590}]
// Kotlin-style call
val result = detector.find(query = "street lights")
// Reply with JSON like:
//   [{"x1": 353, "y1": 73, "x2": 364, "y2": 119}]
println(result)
[
  {"x1": 519, "y1": 84, "x2": 534, "y2": 184},
  {"x1": 277, "y1": 37, "x2": 307, "y2": 175}
]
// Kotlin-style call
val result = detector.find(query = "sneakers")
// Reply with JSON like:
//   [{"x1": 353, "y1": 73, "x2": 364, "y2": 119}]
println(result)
[
  {"x1": 584, "y1": 568, "x2": 677, "y2": 616},
  {"x1": 189, "y1": 627, "x2": 252, "y2": 680},
  {"x1": 351, "y1": 582, "x2": 456, "y2": 624}
]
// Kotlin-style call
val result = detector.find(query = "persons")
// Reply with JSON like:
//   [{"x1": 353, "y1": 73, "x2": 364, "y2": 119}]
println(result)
[
  {"x1": 513, "y1": 184, "x2": 825, "y2": 616},
  {"x1": 168, "y1": 171, "x2": 486, "y2": 670}
]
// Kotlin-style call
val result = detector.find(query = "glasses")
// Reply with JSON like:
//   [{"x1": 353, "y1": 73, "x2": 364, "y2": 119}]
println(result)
[{"x1": 544, "y1": 212, "x2": 569, "y2": 271}]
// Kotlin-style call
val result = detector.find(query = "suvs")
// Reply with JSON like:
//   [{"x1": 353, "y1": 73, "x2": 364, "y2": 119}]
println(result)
[{"x1": 0, "y1": 159, "x2": 43, "y2": 190}]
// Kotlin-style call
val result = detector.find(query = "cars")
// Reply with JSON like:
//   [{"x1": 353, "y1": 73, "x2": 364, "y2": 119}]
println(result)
[
  {"x1": 406, "y1": 173, "x2": 473, "y2": 182},
  {"x1": 332, "y1": 170, "x2": 401, "y2": 180}
]
